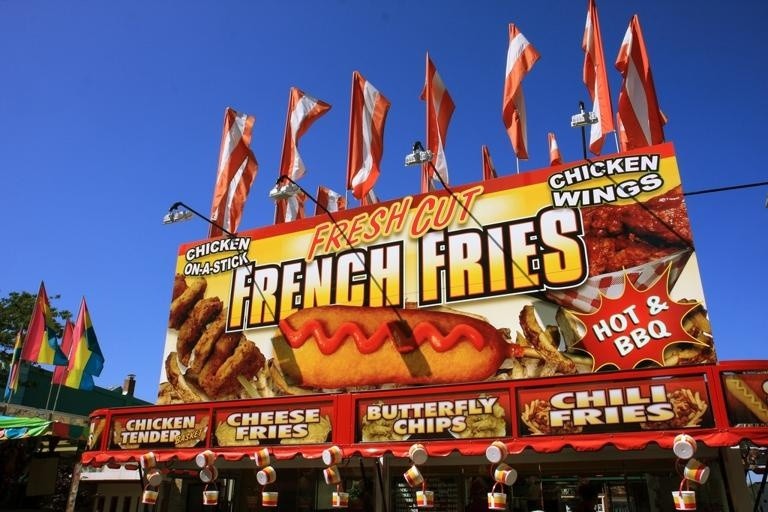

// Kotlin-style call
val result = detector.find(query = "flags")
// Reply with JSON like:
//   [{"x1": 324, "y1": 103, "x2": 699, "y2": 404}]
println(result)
[{"x1": 1, "y1": 279, "x2": 109, "y2": 397}]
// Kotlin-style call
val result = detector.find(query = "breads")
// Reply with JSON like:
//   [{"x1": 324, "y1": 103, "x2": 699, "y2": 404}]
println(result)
[
  {"x1": 271, "y1": 307, "x2": 510, "y2": 388},
  {"x1": 725, "y1": 373, "x2": 768, "y2": 425}
]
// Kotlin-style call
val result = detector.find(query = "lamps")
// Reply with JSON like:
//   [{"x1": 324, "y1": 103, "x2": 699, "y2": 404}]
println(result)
[
  {"x1": 404, "y1": 141, "x2": 450, "y2": 193},
  {"x1": 268, "y1": 174, "x2": 330, "y2": 217},
  {"x1": 571, "y1": 100, "x2": 596, "y2": 161},
  {"x1": 164, "y1": 202, "x2": 238, "y2": 242}
]
